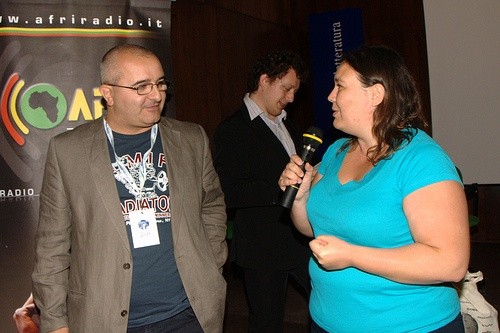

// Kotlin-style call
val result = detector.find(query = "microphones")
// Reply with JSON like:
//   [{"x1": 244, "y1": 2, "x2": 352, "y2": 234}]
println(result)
[{"x1": 280, "y1": 127, "x2": 323, "y2": 209}]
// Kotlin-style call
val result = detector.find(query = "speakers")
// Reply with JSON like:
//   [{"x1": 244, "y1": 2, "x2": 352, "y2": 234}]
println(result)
[{"x1": 308, "y1": 8, "x2": 365, "y2": 134}]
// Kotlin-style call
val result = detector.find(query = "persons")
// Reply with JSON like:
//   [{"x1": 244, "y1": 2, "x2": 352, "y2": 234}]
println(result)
[
  {"x1": 199, "y1": 56, "x2": 324, "y2": 332},
  {"x1": 278, "y1": 50, "x2": 472, "y2": 333},
  {"x1": 29, "y1": 43, "x2": 233, "y2": 332}
]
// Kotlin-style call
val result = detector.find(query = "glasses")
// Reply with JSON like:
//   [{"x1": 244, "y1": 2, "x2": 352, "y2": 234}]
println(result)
[{"x1": 106, "y1": 79, "x2": 168, "y2": 95}]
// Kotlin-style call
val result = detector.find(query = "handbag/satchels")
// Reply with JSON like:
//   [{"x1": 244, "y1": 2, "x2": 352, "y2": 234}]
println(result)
[{"x1": 456, "y1": 269, "x2": 500, "y2": 333}]
